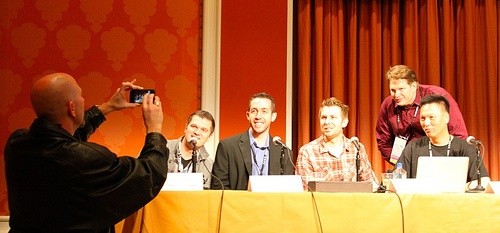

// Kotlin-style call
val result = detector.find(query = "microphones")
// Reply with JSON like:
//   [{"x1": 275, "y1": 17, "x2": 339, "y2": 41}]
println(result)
[
  {"x1": 466, "y1": 136, "x2": 481, "y2": 145},
  {"x1": 188, "y1": 137, "x2": 197, "y2": 146},
  {"x1": 351, "y1": 137, "x2": 361, "y2": 150},
  {"x1": 272, "y1": 136, "x2": 289, "y2": 150}
]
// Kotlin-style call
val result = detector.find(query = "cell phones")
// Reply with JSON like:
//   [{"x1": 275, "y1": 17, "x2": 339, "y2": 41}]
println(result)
[{"x1": 129, "y1": 89, "x2": 156, "y2": 104}]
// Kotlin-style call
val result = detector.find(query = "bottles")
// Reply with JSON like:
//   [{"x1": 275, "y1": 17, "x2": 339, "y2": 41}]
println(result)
[{"x1": 392, "y1": 163, "x2": 407, "y2": 180}]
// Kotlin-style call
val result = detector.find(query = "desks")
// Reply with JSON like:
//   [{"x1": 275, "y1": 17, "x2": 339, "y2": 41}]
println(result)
[{"x1": 114, "y1": 191, "x2": 500, "y2": 233}]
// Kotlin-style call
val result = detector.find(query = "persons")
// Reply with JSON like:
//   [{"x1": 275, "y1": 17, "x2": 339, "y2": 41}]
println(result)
[
  {"x1": 393, "y1": 94, "x2": 491, "y2": 187},
  {"x1": 211, "y1": 93, "x2": 292, "y2": 190},
  {"x1": 137, "y1": 110, "x2": 215, "y2": 188},
  {"x1": 4, "y1": 73, "x2": 169, "y2": 233},
  {"x1": 375, "y1": 64, "x2": 469, "y2": 165},
  {"x1": 294, "y1": 96, "x2": 380, "y2": 191}
]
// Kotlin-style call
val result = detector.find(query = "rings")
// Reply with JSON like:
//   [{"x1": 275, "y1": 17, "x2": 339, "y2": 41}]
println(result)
[{"x1": 156, "y1": 102, "x2": 160, "y2": 106}]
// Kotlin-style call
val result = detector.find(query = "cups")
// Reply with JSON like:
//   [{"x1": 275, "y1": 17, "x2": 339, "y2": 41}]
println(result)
[
  {"x1": 381, "y1": 173, "x2": 394, "y2": 193},
  {"x1": 306, "y1": 171, "x2": 322, "y2": 182}
]
